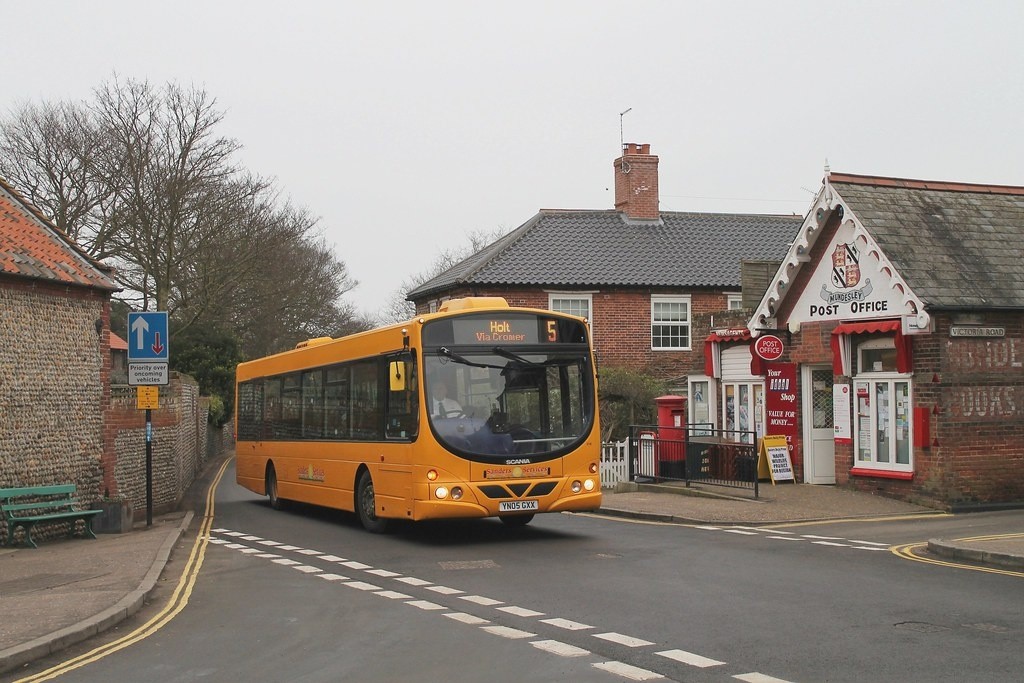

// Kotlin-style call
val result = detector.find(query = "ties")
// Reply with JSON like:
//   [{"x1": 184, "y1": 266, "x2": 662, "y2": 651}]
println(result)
[{"x1": 438, "y1": 402, "x2": 447, "y2": 418}]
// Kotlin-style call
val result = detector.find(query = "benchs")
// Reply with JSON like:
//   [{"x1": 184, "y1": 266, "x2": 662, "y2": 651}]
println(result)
[{"x1": 0, "y1": 483, "x2": 105, "y2": 549}]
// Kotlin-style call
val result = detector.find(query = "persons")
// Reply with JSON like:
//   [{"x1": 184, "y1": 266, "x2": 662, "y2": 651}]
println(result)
[{"x1": 417, "y1": 379, "x2": 467, "y2": 424}]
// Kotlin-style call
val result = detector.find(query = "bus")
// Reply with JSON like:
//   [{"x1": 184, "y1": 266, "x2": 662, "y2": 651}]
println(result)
[{"x1": 234, "y1": 293, "x2": 602, "y2": 533}]
